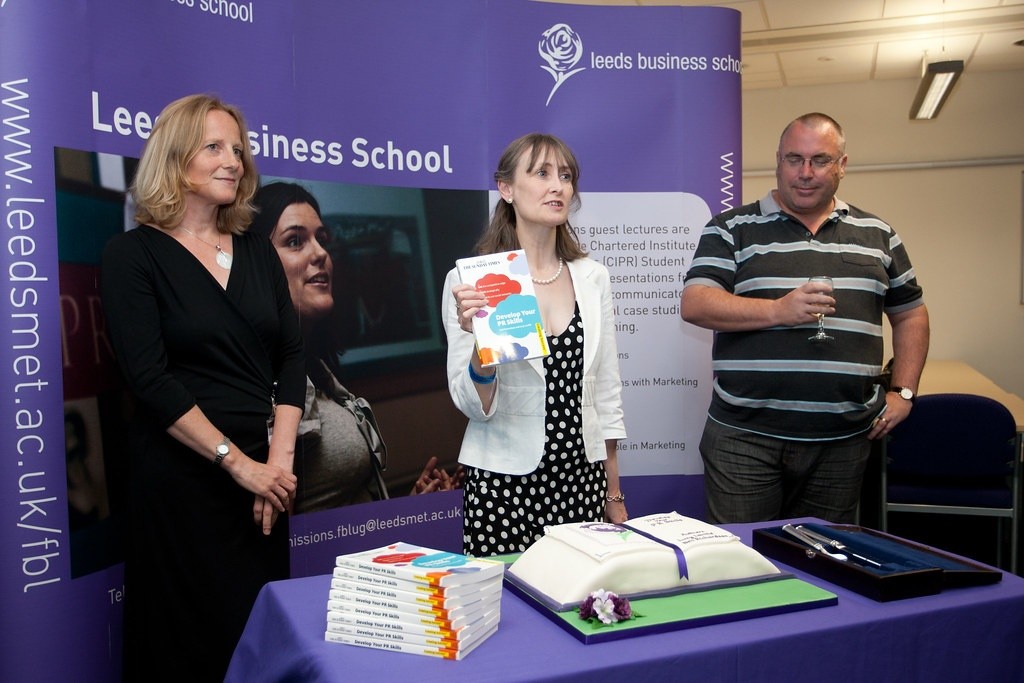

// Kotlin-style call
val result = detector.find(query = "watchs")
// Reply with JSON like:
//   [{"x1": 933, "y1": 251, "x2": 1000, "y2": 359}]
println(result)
[
  {"x1": 886, "y1": 386, "x2": 917, "y2": 405},
  {"x1": 213, "y1": 434, "x2": 232, "y2": 466}
]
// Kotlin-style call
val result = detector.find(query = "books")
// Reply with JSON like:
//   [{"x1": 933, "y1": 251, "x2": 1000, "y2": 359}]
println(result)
[
  {"x1": 453, "y1": 250, "x2": 555, "y2": 369},
  {"x1": 324, "y1": 541, "x2": 508, "y2": 662}
]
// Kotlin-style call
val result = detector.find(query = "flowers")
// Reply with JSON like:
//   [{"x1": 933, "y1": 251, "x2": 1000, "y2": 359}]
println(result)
[{"x1": 573, "y1": 587, "x2": 647, "y2": 628}]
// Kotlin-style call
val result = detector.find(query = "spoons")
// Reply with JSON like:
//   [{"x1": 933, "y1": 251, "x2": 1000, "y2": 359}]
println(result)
[{"x1": 782, "y1": 523, "x2": 848, "y2": 562}]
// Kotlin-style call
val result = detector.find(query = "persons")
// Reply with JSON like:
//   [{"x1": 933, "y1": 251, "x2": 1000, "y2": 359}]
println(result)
[
  {"x1": 679, "y1": 112, "x2": 932, "y2": 523},
  {"x1": 243, "y1": 180, "x2": 464, "y2": 510},
  {"x1": 91, "y1": 93, "x2": 312, "y2": 683},
  {"x1": 441, "y1": 134, "x2": 629, "y2": 559}
]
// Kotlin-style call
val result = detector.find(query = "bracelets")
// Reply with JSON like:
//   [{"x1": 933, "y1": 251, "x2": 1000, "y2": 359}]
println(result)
[
  {"x1": 469, "y1": 361, "x2": 496, "y2": 385},
  {"x1": 606, "y1": 493, "x2": 626, "y2": 503}
]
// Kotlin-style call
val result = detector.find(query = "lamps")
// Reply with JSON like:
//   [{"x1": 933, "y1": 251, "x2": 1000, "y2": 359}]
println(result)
[{"x1": 908, "y1": 46, "x2": 965, "y2": 120}]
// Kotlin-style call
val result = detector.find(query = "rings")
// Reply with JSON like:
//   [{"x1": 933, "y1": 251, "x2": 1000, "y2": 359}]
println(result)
[{"x1": 878, "y1": 417, "x2": 890, "y2": 423}]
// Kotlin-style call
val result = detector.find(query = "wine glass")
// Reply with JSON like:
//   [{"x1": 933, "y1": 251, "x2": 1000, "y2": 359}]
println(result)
[{"x1": 806, "y1": 276, "x2": 836, "y2": 344}]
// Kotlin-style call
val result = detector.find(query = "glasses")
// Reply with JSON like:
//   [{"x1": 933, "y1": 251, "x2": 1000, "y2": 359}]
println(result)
[{"x1": 778, "y1": 152, "x2": 843, "y2": 168}]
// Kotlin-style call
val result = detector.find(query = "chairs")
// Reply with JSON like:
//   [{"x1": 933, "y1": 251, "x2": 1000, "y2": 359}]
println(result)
[{"x1": 881, "y1": 393, "x2": 1024, "y2": 574}]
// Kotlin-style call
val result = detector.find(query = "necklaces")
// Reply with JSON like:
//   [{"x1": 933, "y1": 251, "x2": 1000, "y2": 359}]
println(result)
[
  {"x1": 526, "y1": 256, "x2": 565, "y2": 284},
  {"x1": 173, "y1": 223, "x2": 234, "y2": 271}
]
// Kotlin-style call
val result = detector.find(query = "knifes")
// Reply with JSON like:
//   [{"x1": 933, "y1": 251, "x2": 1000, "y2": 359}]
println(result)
[{"x1": 796, "y1": 524, "x2": 881, "y2": 568}]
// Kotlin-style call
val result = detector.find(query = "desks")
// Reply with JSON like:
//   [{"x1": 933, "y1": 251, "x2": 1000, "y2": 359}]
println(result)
[{"x1": 223, "y1": 515, "x2": 1024, "y2": 683}]
